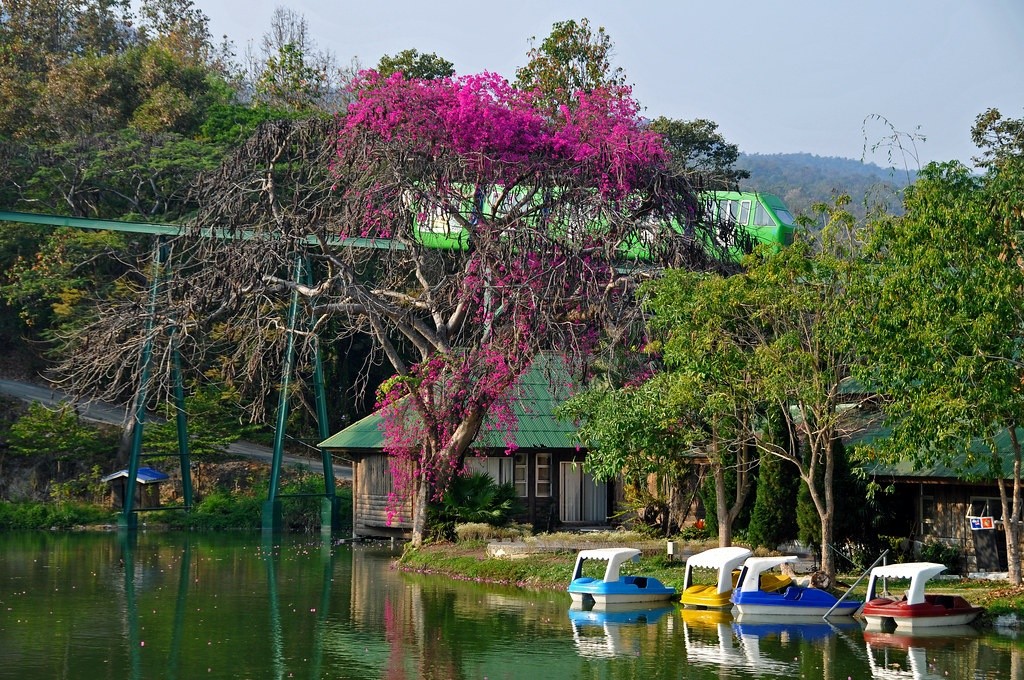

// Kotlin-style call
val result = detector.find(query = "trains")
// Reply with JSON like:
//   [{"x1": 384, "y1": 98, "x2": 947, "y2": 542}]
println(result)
[{"x1": 413, "y1": 180, "x2": 797, "y2": 260}]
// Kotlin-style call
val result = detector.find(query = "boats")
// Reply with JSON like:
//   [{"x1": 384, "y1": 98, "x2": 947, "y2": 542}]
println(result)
[
  {"x1": 863, "y1": 624, "x2": 979, "y2": 680},
  {"x1": 728, "y1": 556, "x2": 863, "y2": 616},
  {"x1": 681, "y1": 610, "x2": 737, "y2": 655},
  {"x1": 567, "y1": 547, "x2": 675, "y2": 604},
  {"x1": 863, "y1": 562, "x2": 984, "y2": 627},
  {"x1": 568, "y1": 602, "x2": 676, "y2": 657},
  {"x1": 679, "y1": 547, "x2": 795, "y2": 609},
  {"x1": 731, "y1": 615, "x2": 858, "y2": 663}
]
